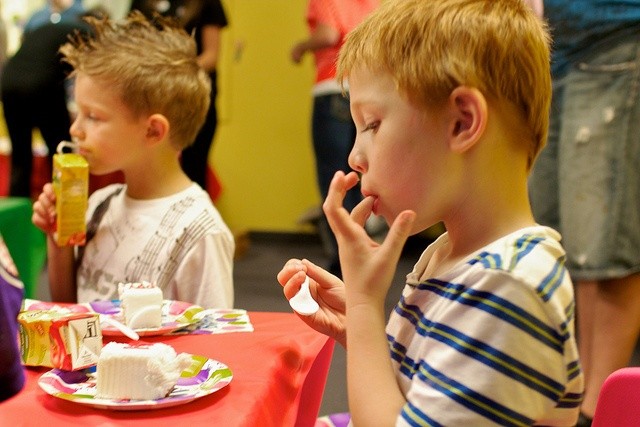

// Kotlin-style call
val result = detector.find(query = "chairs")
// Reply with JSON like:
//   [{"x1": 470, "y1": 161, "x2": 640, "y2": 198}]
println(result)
[{"x1": 590, "y1": 367, "x2": 640, "y2": 427}]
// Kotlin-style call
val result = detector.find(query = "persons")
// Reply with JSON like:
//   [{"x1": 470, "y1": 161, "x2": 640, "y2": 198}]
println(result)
[
  {"x1": 275, "y1": 1, "x2": 586, "y2": 426},
  {"x1": 289, "y1": 1, "x2": 403, "y2": 287},
  {"x1": 526, "y1": 1, "x2": 640, "y2": 424},
  {"x1": 31, "y1": 11, "x2": 237, "y2": 309},
  {"x1": 127, "y1": 0, "x2": 229, "y2": 191},
  {"x1": 1, "y1": 1, "x2": 108, "y2": 199}
]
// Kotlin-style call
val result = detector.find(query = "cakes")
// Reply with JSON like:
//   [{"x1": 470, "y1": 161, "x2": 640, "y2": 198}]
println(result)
[
  {"x1": 96, "y1": 342, "x2": 179, "y2": 399},
  {"x1": 117, "y1": 282, "x2": 163, "y2": 330}
]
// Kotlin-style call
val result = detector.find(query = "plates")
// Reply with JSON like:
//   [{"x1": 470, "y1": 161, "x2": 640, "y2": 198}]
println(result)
[
  {"x1": 37, "y1": 353, "x2": 233, "y2": 412},
  {"x1": 78, "y1": 299, "x2": 206, "y2": 337}
]
